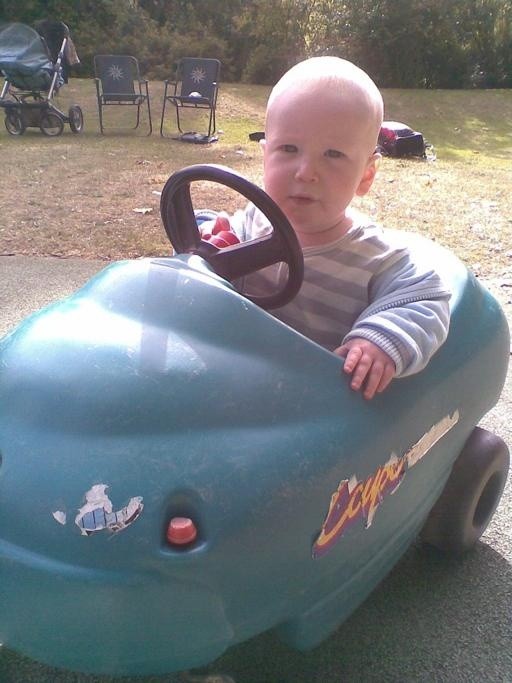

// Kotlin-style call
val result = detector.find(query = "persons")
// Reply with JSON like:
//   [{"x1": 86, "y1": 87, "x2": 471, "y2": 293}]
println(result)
[{"x1": 192, "y1": 56, "x2": 450, "y2": 401}]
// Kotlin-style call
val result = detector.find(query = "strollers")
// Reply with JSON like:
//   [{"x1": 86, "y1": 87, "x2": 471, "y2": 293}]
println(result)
[{"x1": 0, "y1": 17, "x2": 84, "y2": 135}]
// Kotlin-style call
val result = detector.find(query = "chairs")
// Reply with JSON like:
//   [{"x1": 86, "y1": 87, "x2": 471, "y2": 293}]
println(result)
[
  {"x1": 92, "y1": 53, "x2": 154, "y2": 137},
  {"x1": 160, "y1": 53, "x2": 221, "y2": 139}
]
